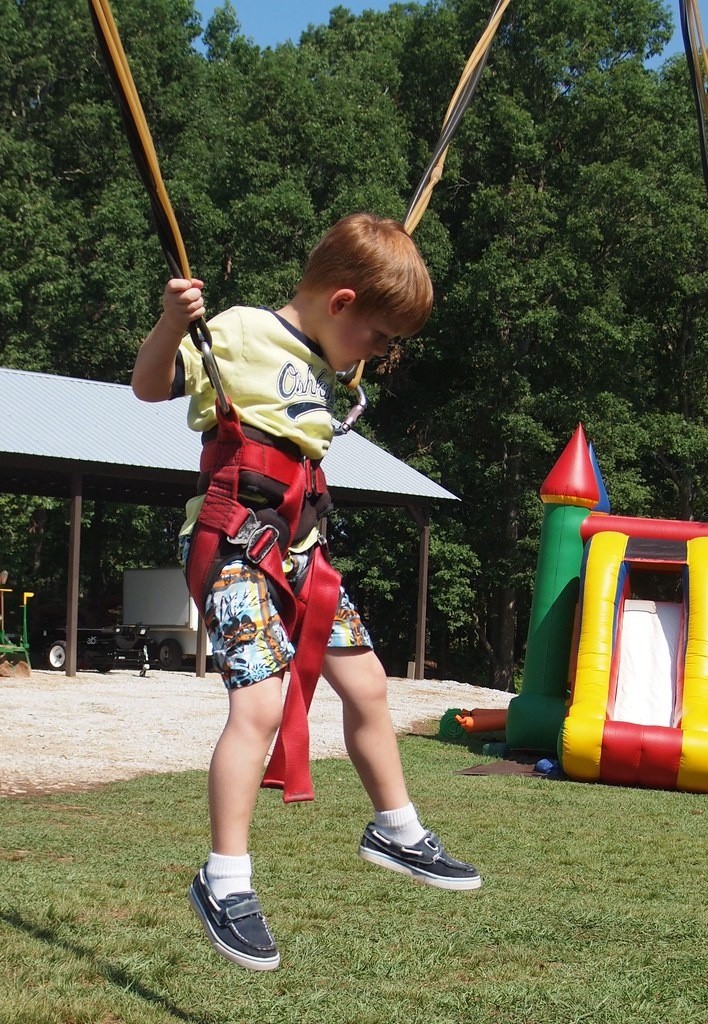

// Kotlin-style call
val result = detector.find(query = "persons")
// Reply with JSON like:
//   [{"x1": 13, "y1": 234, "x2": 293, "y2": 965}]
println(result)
[{"x1": 132, "y1": 212, "x2": 482, "y2": 970}]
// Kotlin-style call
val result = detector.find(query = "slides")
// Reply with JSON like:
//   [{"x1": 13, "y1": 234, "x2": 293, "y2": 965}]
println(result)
[{"x1": 557, "y1": 597, "x2": 708, "y2": 794}]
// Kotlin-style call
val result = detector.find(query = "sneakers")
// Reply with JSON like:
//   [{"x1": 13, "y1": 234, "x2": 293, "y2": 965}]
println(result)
[
  {"x1": 189, "y1": 861, "x2": 281, "y2": 970},
  {"x1": 356, "y1": 822, "x2": 482, "y2": 890}
]
